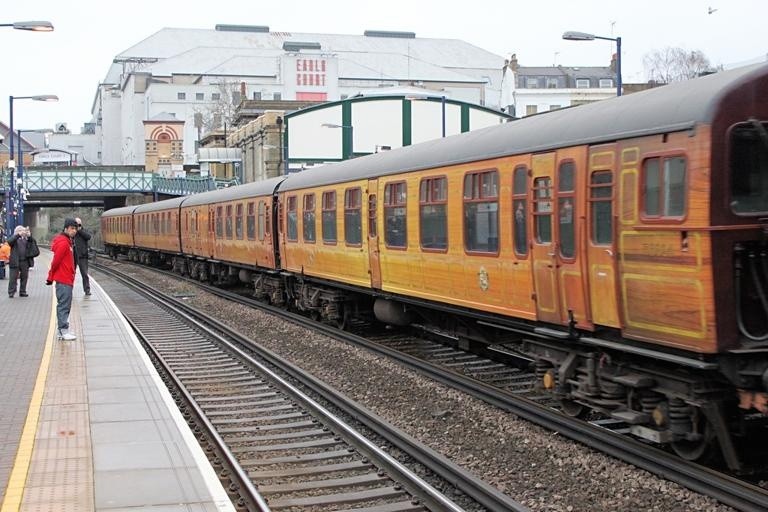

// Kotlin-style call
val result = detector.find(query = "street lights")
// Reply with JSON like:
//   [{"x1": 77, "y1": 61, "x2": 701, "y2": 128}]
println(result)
[
  {"x1": 562, "y1": 29, "x2": 623, "y2": 98},
  {"x1": 6, "y1": 93, "x2": 60, "y2": 237}
]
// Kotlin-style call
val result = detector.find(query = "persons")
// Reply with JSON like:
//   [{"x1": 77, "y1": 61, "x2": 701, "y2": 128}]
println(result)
[
  {"x1": 45, "y1": 217, "x2": 79, "y2": 341},
  {"x1": 6, "y1": 225, "x2": 38, "y2": 298},
  {"x1": 71, "y1": 217, "x2": 92, "y2": 295},
  {"x1": 0, "y1": 236, "x2": 12, "y2": 279}
]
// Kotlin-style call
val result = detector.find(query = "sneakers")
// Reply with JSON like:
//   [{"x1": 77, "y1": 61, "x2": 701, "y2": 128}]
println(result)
[
  {"x1": 20, "y1": 292, "x2": 28, "y2": 296},
  {"x1": 86, "y1": 288, "x2": 91, "y2": 295},
  {"x1": 9, "y1": 292, "x2": 13, "y2": 297}
]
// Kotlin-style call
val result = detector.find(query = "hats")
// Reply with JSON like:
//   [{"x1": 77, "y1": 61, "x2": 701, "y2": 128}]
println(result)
[{"x1": 64, "y1": 217, "x2": 79, "y2": 227}]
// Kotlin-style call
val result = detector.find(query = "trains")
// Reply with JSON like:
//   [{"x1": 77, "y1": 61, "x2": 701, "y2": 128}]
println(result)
[{"x1": 98, "y1": 58, "x2": 768, "y2": 474}]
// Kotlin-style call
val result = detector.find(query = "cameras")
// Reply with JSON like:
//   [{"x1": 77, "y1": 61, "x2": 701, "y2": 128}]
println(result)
[{"x1": 78, "y1": 224, "x2": 82, "y2": 227}]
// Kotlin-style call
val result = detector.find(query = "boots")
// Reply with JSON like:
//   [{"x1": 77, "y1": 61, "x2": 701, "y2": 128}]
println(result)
[{"x1": 57, "y1": 328, "x2": 77, "y2": 341}]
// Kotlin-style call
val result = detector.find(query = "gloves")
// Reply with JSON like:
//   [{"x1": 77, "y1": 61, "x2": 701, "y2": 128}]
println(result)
[{"x1": 45, "y1": 280, "x2": 52, "y2": 285}]
